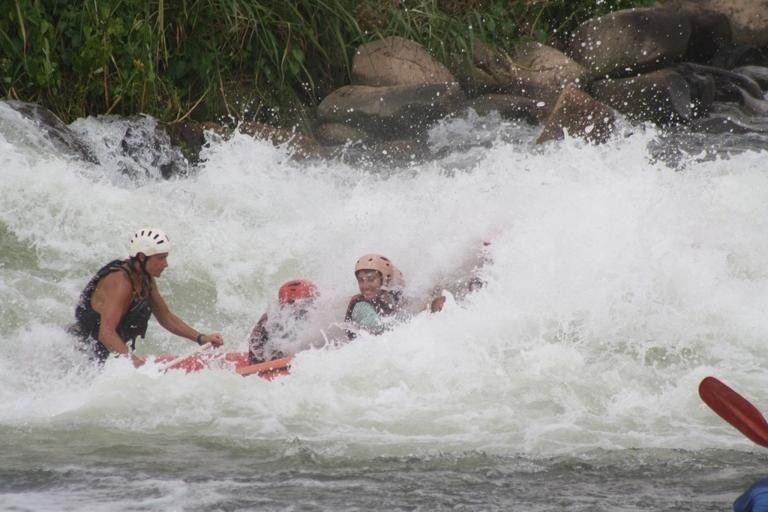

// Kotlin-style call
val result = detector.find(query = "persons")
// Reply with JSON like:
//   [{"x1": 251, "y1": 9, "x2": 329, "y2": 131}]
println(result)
[
  {"x1": 342, "y1": 252, "x2": 409, "y2": 343},
  {"x1": 247, "y1": 277, "x2": 324, "y2": 367},
  {"x1": 63, "y1": 229, "x2": 226, "y2": 372},
  {"x1": 381, "y1": 266, "x2": 449, "y2": 323}
]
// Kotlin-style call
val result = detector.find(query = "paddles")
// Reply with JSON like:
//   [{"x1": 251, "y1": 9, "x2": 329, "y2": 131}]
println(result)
[{"x1": 698, "y1": 376, "x2": 764, "y2": 446}]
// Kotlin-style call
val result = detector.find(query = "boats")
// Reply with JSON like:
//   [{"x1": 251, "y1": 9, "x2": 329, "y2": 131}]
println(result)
[
  {"x1": 734, "y1": 476, "x2": 768, "y2": 512},
  {"x1": 143, "y1": 350, "x2": 294, "y2": 383}
]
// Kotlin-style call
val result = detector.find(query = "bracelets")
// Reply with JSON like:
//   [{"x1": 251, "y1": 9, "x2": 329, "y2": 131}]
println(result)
[{"x1": 197, "y1": 334, "x2": 206, "y2": 346}]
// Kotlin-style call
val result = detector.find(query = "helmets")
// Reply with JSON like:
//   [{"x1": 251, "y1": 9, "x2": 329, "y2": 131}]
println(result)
[
  {"x1": 278, "y1": 278, "x2": 322, "y2": 304},
  {"x1": 355, "y1": 253, "x2": 393, "y2": 280},
  {"x1": 129, "y1": 228, "x2": 172, "y2": 258}
]
